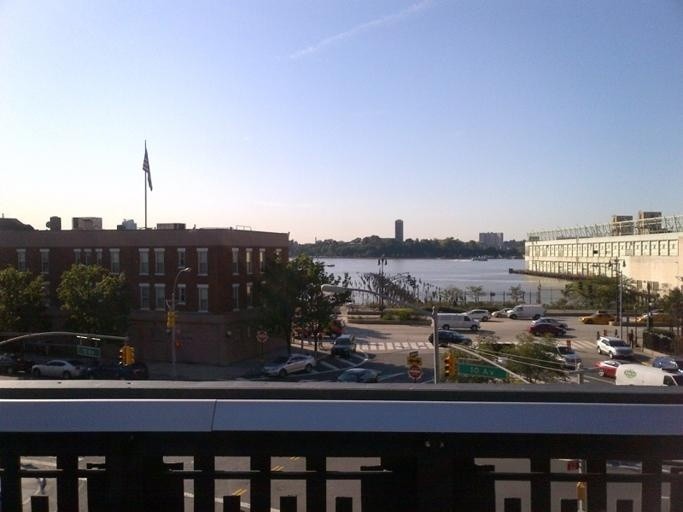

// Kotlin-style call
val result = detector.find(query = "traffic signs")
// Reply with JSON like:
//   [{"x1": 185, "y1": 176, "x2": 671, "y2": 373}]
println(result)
[
  {"x1": 455, "y1": 362, "x2": 508, "y2": 380},
  {"x1": 74, "y1": 345, "x2": 102, "y2": 359},
  {"x1": 409, "y1": 351, "x2": 419, "y2": 358}
]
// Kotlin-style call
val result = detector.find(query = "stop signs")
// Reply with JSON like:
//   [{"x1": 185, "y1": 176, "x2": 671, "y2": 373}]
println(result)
[
  {"x1": 408, "y1": 364, "x2": 423, "y2": 380},
  {"x1": 256, "y1": 331, "x2": 269, "y2": 343}
]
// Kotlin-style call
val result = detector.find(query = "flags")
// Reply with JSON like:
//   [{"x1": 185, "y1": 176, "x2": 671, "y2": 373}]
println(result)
[{"x1": 142, "y1": 142, "x2": 152, "y2": 191}]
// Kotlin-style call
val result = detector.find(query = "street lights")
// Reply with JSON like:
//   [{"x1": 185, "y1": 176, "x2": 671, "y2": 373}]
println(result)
[
  {"x1": 377, "y1": 254, "x2": 388, "y2": 317},
  {"x1": 608, "y1": 257, "x2": 627, "y2": 324},
  {"x1": 643, "y1": 280, "x2": 656, "y2": 330},
  {"x1": 168, "y1": 266, "x2": 192, "y2": 365},
  {"x1": 321, "y1": 263, "x2": 338, "y2": 268},
  {"x1": 318, "y1": 282, "x2": 439, "y2": 383}
]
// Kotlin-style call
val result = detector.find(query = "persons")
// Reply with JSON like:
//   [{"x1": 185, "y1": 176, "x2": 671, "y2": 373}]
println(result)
[
  {"x1": 16, "y1": 352, "x2": 32, "y2": 370},
  {"x1": 628, "y1": 329, "x2": 633, "y2": 348}
]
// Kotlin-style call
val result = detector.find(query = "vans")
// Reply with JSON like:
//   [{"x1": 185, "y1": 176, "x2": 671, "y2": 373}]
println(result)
[{"x1": 612, "y1": 363, "x2": 682, "y2": 387}]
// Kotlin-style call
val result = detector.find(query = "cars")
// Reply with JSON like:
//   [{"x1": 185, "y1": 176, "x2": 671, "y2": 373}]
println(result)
[
  {"x1": 490, "y1": 308, "x2": 511, "y2": 318},
  {"x1": 29, "y1": 356, "x2": 87, "y2": 379},
  {"x1": 544, "y1": 345, "x2": 583, "y2": 370},
  {"x1": 460, "y1": 309, "x2": 490, "y2": 322},
  {"x1": 530, "y1": 316, "x2": 567, "y2": 328},
  {"x1": 426, "y1": 330, "x2": 473, "y2": 349},
  {"x1": 0, "y1": 351, "x2": 35, "y2": 376},
  {"x1": 575, "y1": 308, "x2": 682, "y2": 379},
  {"x1": 262, "y1": 353, "x2": 316, "y2": 377},
  {"x1": 527, "y1": 322, "x2": 568, "y2": 339},
  {"x1": 333, "y1": 368, "x2": 382, "y2": 384}
]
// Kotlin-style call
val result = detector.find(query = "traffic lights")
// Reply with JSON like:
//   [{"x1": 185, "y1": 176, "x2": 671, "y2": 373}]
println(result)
[
  {"x1": 118, "y1": 347, "x2": 126, "y2": 367},
  {"x1": 126, "y1": 345, "x2": 135, "y2": 366},
  {"x1": 442, "y1": 354, "x2": 452, "y2": 377},
  {"x1": 167, "y1": 312, "x2": 175, "y2": 328}
]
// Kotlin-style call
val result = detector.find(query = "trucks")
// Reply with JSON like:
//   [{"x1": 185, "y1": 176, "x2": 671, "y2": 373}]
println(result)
[
  {"x1": 428, "y1": 313, "x2": 481, "y2": 331},
  {"x1": 506, "y1": 303, "x2": 546, "y2": 321}
]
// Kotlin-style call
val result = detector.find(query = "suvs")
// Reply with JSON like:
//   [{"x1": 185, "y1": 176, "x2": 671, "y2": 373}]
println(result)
[
  {"x1": 328, "y1": 334, "x2": 357, "y2": 359},
  {"x1": 294, "y1": 317, "x2": 347, "y2": 340}
]
